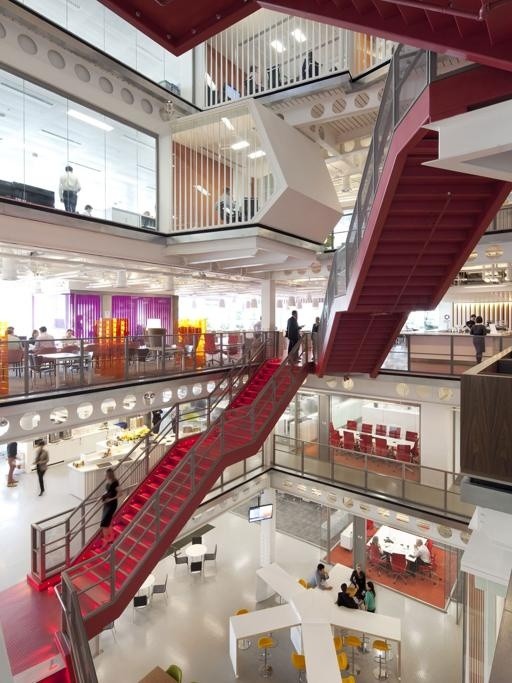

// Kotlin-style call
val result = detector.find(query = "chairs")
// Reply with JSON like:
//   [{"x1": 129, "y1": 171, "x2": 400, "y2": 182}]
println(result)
[{"x1": 323, "y1": 415, "x2": 423, "y2": 475}]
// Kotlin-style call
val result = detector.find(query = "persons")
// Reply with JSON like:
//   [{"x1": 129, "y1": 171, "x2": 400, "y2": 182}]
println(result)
[
  {"x1": 463, "y1": 313, "x2": 478, "y2": 327},
  {"x1": 82, "y1": 204, "x2": 95, "y2": 216},
  {"x1": 61, "y1": 328, "x2": 79, "y2": 366},
  {"x1": 100, "y1": 469, "x2": 126, "y2": 550},
  {"x1": 33, "y1": 440, "x2": 51, "y2": 496},
  {"x1": 371, "y1": 536, "x2": 391, "y2": 569},
  {"x1": 336, "y1": 583, "x2": 360, "y2": 609},
  {"x1": 248, "y1": 65, "x2": 264, "y2": 91},
  {"x1": 301, "y1": 51, "x2": 320, "y2": 79},
  {"x1": 252, "y1": 315, "x2": 263, "y2": 339},
  {"x1": 219, "y1": 187, "x2": 241, "y2": 222},
  {"x1": 310, "y1": 316, "x2": 320, "y2": 360},
  {"x1": 29, "y1": 327, "x2": 58, "y2": 371},
  {"x1": 308, "y1": 563, "x2": 332, "y2": 591},
  {"x1": 469, "y1": 316, "x2": 491, "y2": 365},
  {"x1": 284, "y1": 309, "x2": 305, "y2": 364},
  {"x1": 7, "y1": 440, "x2": 24, "y2": 487},
  {"x1": 359, "y1": 580, "x2": 376, "y2": 612},
  {"x1": 151, "y1": 409, "x2": 164, "y2": 432},
  {"x1": 58, "y1": 164, "x2": 82, "y2": 212},
  {"x1": 408, "y1": 538, "x2": 432, "y2": 576},
  {"x1": 28, "y1": 328, "x2": 39, "y2": 365},
  {"x1": 350, "y1": 563, "x2": 366, "y2": 600},
  {"x1": 5, "y1": 328, "x2": 25, "y2": 354},
  {"x1": 7, "y1": 325, "x2": 20, "y2": 338}
]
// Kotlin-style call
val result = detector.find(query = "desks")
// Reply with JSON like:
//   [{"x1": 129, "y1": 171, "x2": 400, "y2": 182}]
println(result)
[
  {"x1": 0, "y1": 178, "x2": 156, "y2": 230},
  {"x1": 65, "y1": 397, "x2": 320, "y2": 506}
]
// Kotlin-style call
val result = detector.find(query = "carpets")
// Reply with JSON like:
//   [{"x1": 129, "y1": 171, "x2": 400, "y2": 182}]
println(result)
[{"x1": 289, "y1": 429, "x2": 422, "y2": 485}]
